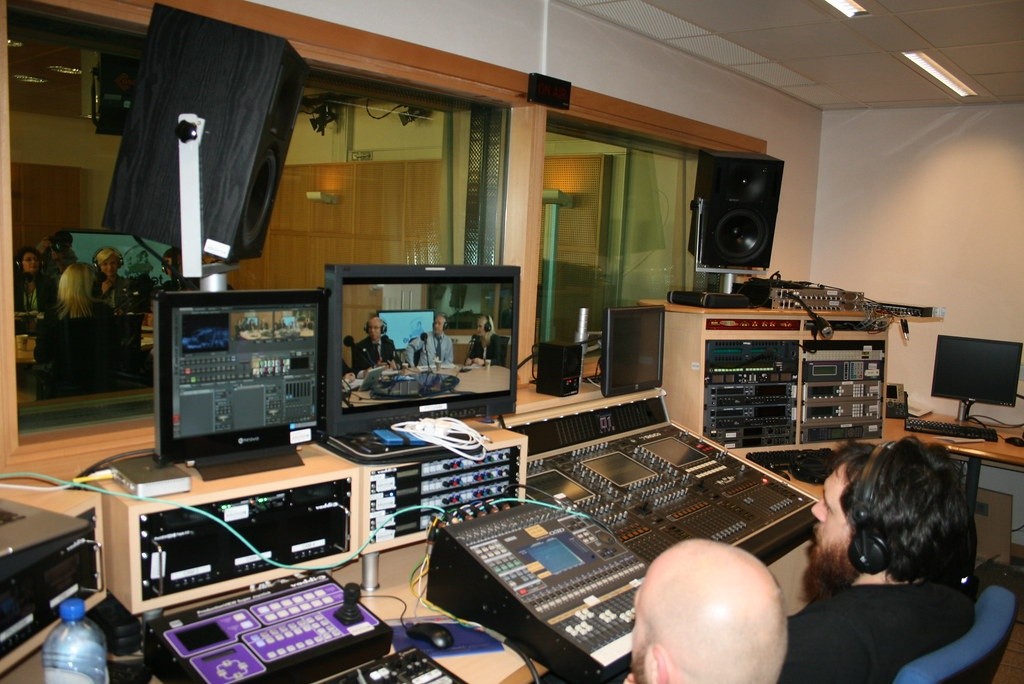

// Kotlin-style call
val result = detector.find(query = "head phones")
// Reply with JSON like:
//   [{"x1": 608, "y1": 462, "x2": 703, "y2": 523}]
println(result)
[
  {"x1": 92, "y1": 246, "x2": 125, "y2": 276},
  {"x1": 483, "y1": 314, "x2": 492, "y2": 333},
  {"x1": 364, "y1": 319, "x2": 388, "y2": 334},
  {"x1": 432, "y1": 313, "x2": 448, "y2": 330},
  {"x1": 15, "y1": 246, "x2": 45, "y2": 274},
  {"x1": 846, "y1": 440, "x2": 898, "y2": 575}
]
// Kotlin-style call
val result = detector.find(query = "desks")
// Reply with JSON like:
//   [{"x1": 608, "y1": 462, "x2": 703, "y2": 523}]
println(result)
[
  {"x1": 880, "y1": 412, "x2": 1024, "y2": 513},
  {"x1": 639, "y1": 299, "x2": 945, "y2": 449}
]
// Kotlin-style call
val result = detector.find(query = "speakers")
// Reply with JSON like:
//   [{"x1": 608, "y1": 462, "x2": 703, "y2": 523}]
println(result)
[
  {"x1": 688, "y1": 149, "x2": 784, "y2": 268},
  {"x1": 100, "y1": 2, "x2": 308, "y2": 261}
]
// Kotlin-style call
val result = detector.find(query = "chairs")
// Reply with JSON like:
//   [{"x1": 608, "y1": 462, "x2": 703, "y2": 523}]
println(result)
[{"x1": 891, "y1": 586, "x2": 1017, "y2": 684}]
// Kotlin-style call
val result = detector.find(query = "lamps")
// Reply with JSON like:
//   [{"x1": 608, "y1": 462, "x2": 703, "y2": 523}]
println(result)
[
  {"x1": 398, "y1": 106, "x2": 427, "y2": 127},
  {"x1": 902, "y1": 51, "x2": 977, "y2": 97},
  {"x1": 309, "y1": 103, "x2": 336, "y2": 136}
]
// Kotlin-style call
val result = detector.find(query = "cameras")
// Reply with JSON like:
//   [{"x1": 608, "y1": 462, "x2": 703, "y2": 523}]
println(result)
[{"x1": 48, "y1": 238, "x2": 70, "y2": 255}]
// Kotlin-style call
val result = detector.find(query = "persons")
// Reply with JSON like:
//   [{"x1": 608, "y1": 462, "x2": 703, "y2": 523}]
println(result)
[
  {"x1": 13, "y1": 231, "x2": 234, "y2": 401},
  {"x1": 352, "y1": 317, "x2": 403, "y2": 379},
  {"x1": 776, "y1": 435, "x2": 974, "y2": 684},
  {"x1": 342, "y1": 358, "x2": 355, "y2": 383},
  {"x1": 464, "y1": 315, "x2": 505, "y2": 367},
  {"x1": 406, "y1": 312, "x2": 453, "y2": 368},
  {"x1": 622, "y1": 539, "x2": 788, "y2": 684}
]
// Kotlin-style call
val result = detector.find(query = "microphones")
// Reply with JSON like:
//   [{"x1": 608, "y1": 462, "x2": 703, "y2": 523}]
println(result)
[
  {"x1": 420, "y1": 332, "x2": 428, "y2": 343},
  {"x1": 470, "y1": 334, "x2": 478, "y2": 343},
  {"x1": 344, "y1": 335, "x2": 368, "y2": 352}
]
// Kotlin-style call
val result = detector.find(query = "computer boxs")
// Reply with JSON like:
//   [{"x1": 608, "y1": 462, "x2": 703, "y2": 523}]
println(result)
[{"x1": 536, "y1": 340, "x2": 582, "y2": 397}]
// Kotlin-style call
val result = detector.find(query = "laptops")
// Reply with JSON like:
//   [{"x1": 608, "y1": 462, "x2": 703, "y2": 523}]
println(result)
[{"x1": 345, "y1": 364, "x2": 384, "y2": 392}]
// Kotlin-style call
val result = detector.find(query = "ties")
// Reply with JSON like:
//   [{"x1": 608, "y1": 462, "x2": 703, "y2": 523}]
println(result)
[
  {"x1": 435, "y1": 336, "x2": 443, "y2": 361},
  {"x1": 373, "y1": 343, "x2": 382, "y2": 365}
]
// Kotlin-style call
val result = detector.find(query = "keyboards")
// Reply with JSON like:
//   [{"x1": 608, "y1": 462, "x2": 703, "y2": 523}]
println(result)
[
  {"x1": 904, "y1": 418, "x2": 998, "y2": 443},
  {"x1": 745, "y1": 448, "x2": 835, "y2": 470}
]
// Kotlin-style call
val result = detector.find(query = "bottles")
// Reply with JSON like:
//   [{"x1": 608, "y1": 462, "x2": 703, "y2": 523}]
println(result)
[{"x1": 42, "y1": 599, "x2": 109, "y2": 684}]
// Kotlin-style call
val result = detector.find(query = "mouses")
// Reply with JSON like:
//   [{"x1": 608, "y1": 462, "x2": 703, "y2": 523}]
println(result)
[
  {"x1": 406, "y1": 623, "x2": 456, "y2": 648},
  {"x1": 1005, "y1": 437, "x2": 1024, "y2": 447},
  {"x1": 790, "y1": 453, "x2": 834, "y2": 484}
]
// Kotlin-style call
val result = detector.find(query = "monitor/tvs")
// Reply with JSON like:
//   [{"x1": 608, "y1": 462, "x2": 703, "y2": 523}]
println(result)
[
  {"x1": 152, "y1": 288, "x2": 329, "y2": 480},
  {"x1": 324, "y1": 263, "x2": 521, "y2": 462},
  {"x1": 931, "y1": 335, "x2": 1023, "y2": 407},
  {"x1": 600, "y1": 306, "x2": 665, "y2": 397},
  {"x1": 376, "y1": 309, "x2": 435, "y2": 351}
]
